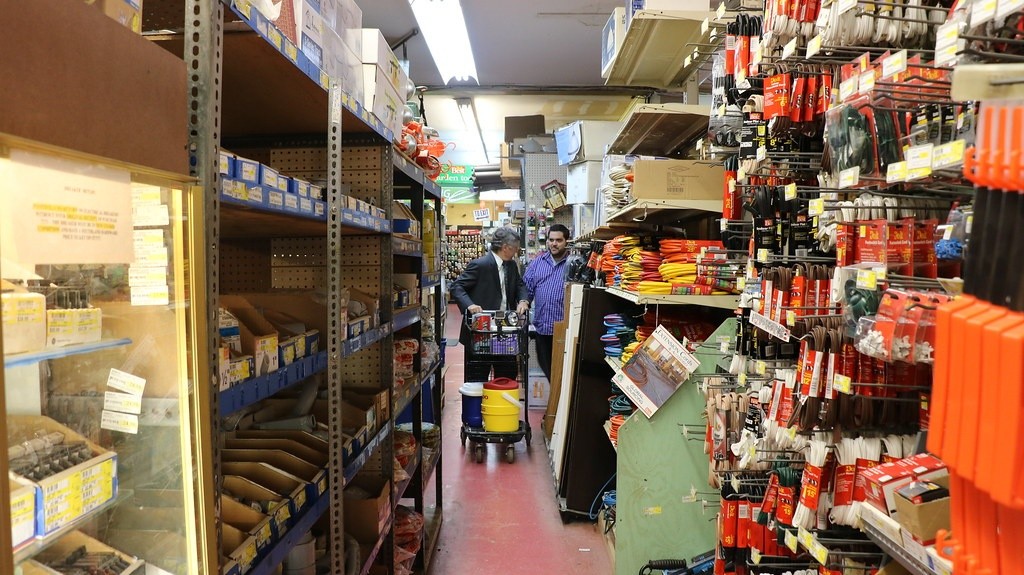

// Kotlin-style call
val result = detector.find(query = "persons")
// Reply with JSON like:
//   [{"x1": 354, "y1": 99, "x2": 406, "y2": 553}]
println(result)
[
  {"x1": 522, "y1": 224, "x2": 571, "y2": 383},
  {"x1": 449, "y1": 228, "x2": 531, "y2": 444}
]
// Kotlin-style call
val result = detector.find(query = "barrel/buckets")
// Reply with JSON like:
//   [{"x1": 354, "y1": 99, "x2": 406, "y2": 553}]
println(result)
[
  {"x1": 458, "y1": 382, "x2": 483, "y2": 426},
  {"x1": 481, "y1": 377, "x2": 522, "y2": 432}
]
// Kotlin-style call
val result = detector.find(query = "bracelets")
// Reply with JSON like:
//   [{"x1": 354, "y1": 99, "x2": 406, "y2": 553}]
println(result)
[{"x1": 519, "y1": 300, "x2": 529, "y2": 305}]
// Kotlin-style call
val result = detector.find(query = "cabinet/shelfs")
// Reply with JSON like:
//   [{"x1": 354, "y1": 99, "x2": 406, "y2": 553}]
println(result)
[
  {"x1": 0, "y1": 0, "x2": 210, "y2": 575},
  {"x1": 266, "y1": 141, "x2": 445, "y2": 575},
  {"x1": 132, "y1": 0, "x2": 395, "y2": 575},
  {"x1": 543, "y1": 0, "x2": 1024, "y2": 575},
  {"x1": 423, "y1": 214, "x2": 446, "y2": 412},
  {"x1": 445, "y1": 146, "x2": 568, "y2": 307}
]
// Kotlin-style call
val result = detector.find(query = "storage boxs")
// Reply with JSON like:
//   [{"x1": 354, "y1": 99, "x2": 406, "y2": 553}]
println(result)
[
  {"x1": 217, "y1": 151, "x2": 386, "y2": 219},
  {"x1": 251, "y1": 2, "x2": 410, "y2": 136},
  {"x1": 219, "y1": 288, "x2": 383, "y2": 391},
  {"x1": 559, "y1": 121, "x2": 622, "y2": 164},
  {"x1": 566, "y1": 162, "x2": 606, "y2": 204},
  {"x1": 501, "y1": 135, "x2": 556, "y2": 190},
  {"x1": 628, "y1": 159, "x2": 724, "y2": 203},
  {"x1": 600, "y1": 7, "x2": 627, "y2": 78},
  {"x1": 219, "y1": 386, "x2": 390, "y2": 575},
  {"x1": 572, "y1": 204, "x2": 595, "y2": 238},
  {"x1": 627, "y1": 0, "x2": 711, "y2": 28},
  {"x1": 0, "y1": 294, "x2": 101, "y2": 359},
  {"x1": 9, "y1": 414, "x2": 146, "y2": 575}
]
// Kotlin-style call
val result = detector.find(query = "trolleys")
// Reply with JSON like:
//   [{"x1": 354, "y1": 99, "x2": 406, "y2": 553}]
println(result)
[{"x1": 461, "y1": 308, "x2": 531, "y2": 464}]
